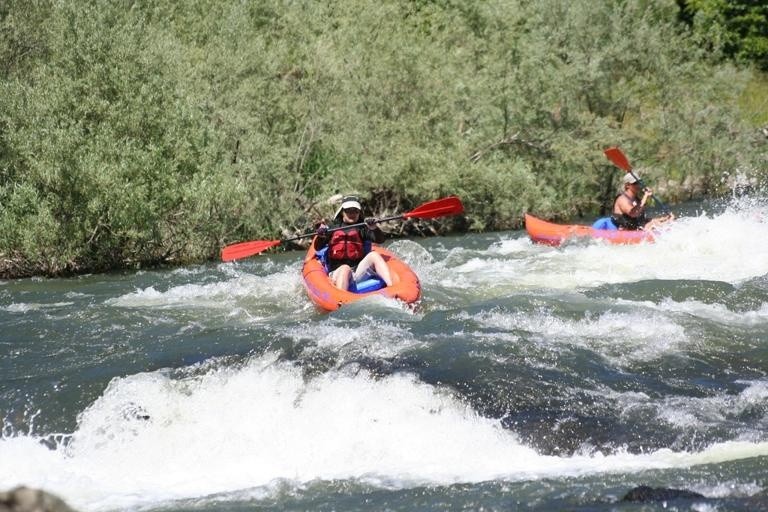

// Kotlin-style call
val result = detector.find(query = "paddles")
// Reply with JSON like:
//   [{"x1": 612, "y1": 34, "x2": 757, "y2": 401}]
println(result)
[
  {"x1": 604, "y1": 148, "x2": 672, "y2": 217},
  {"x1": 221, "y1": 196, "x2": 463, "y2": 262}
]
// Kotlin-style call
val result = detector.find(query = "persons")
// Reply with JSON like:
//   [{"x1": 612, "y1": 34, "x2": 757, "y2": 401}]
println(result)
[
  {"x1": 314, "y1": 195, "x2": 395, "y2": 292},
  {"x1": 612, "y1": 173, "x2": 675, "y2": 232}
]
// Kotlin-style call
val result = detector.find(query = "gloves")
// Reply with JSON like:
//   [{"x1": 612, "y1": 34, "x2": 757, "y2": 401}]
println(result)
[
  {"x1": 364, "y1": 217, "x2": 379, "y2": 230},
  {"x1": 317, "y1": 224, "x2": 330, "y2": 238}
]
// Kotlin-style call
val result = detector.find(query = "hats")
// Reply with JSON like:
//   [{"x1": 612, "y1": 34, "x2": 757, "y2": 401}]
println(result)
[
  {"x1": 624, "y1": 172, "x2": 642, "y2": 184},
  {"x1": 342, "y1": 196, "x2": 362, "y2": 210}
]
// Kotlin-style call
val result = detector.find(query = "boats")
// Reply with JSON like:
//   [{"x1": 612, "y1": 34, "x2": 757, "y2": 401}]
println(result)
[
  {"x1": 303, "y1": 221, "x2": 423, "y2": 313},
  {"x1": 523, "y1": 207, "x2": 678, "y2": 247}
]
